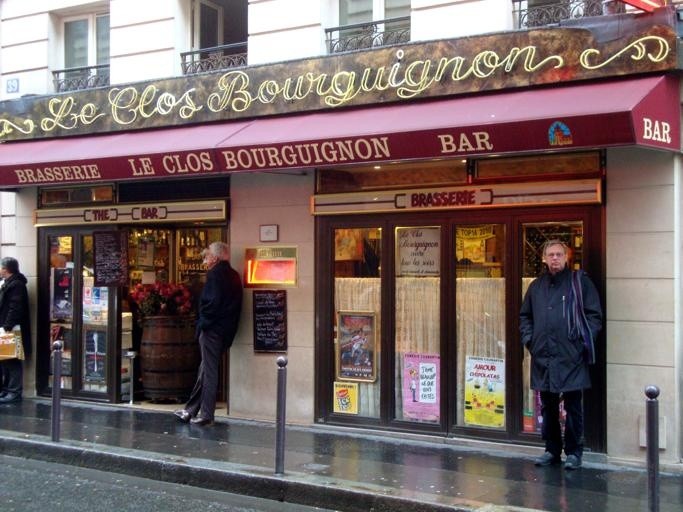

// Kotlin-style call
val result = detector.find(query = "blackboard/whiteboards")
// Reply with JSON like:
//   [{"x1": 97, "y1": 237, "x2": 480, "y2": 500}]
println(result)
[
  {"x1": 253, "y1": 290, "x2": 287, "y2": 353},
  {"x1": 93, "y1": 230, "x2": 129, "y2": 287}
]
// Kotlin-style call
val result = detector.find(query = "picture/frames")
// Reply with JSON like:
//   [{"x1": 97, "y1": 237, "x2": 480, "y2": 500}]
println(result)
[
  {"x1": 395, "y1": 225, "x2": 441, "y2": 277},
  {"x1": 336, "y1": 309, "x2": 377, "y2": 383},
  {"x1": 259, "y1": 223, "x2": 278, "y2": 242}
]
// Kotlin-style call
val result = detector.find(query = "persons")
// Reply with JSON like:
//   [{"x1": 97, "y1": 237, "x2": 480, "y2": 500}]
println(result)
[
  {"x1": 518, "y1": 239, "x2": 604, "y2": 470},
  {"x1": 341, "y1": 331, "x2": 367, "y2": 365},
  {"x1": 0, "y1": 257, "x2": 32, "y2": 404},
  {"x1": 173, "y1": 241, "x2": 244, "y2": 425}
]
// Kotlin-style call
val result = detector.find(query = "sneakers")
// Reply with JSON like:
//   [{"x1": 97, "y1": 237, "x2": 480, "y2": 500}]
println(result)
[
  {"x1": 563, "y1": 455, "x2": 582, "y2": 469},
  {"x1": 535, "y1": 451, "x2": 561, "y2": 466}
]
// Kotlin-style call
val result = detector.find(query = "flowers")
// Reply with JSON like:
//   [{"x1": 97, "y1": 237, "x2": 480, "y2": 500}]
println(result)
[{"x1": 130, "y1": 280, "x2": 193, "y2": 314}]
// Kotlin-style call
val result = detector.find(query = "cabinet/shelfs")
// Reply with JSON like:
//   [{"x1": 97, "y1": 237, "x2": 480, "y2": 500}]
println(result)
[
  {"x1": 51, "y1": 312, "x2": 134, "y2": 401},
  {"x1": 176, "y1": 229, "x2": 209, "y2": 284},
  {"x1": 129, "y1": 243, "x2": 169, "y2": 284}
]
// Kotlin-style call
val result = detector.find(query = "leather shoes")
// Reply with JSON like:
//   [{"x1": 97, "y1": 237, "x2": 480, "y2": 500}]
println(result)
[
  {"x1": 190, "y1": 418, "x2": 214, "y2": 427},
  {"x1": 173, "y1": 410, "x2": 191, "y2": 423},
  {"x1": 0, "y1": 392, "x2": 22, "y2": 402}
]
